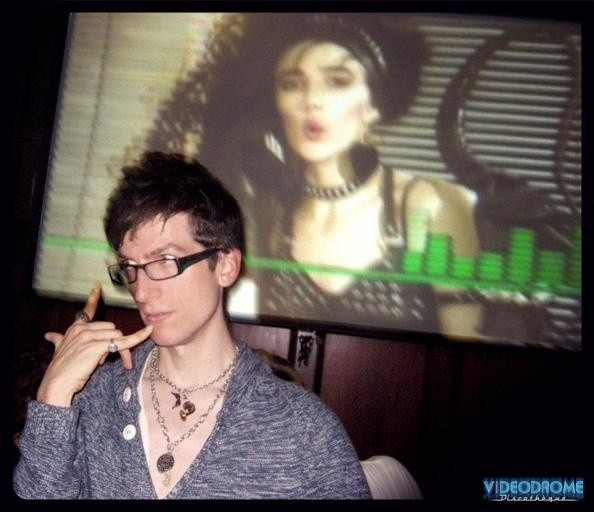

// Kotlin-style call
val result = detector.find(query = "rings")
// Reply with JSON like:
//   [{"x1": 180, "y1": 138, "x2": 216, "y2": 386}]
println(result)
[
  {"x1": 107, "y1": 337, "x2": 118, "y2": 354},
  {"x1": 74, "y1": 309, "x2": 91, "y2": 323}
]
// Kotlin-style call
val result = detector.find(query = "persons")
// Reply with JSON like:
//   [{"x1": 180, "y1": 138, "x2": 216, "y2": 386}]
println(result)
[
  {"x1": 126, "y1": 6, "x2": 490, "y2": 342},
  {"x1": 0, "y1": 145, "x2": 376, "y2": 501}
]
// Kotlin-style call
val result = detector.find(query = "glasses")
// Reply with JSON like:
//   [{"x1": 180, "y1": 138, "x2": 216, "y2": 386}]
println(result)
[{"x1": 108, "y1": 248, "x2": 217, "y2": 284}]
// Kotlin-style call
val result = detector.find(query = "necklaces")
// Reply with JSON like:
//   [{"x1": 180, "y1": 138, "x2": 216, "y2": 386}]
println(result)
[
  {"x1": 148, "y1": 344, "x2": 241, "y2": 420},
  {"x1": 286, "y1": 167, "x2": 375, "y2": 203},
  {"x1": 145, "y1": 343, "x2": 242, "y2": 485}
]
